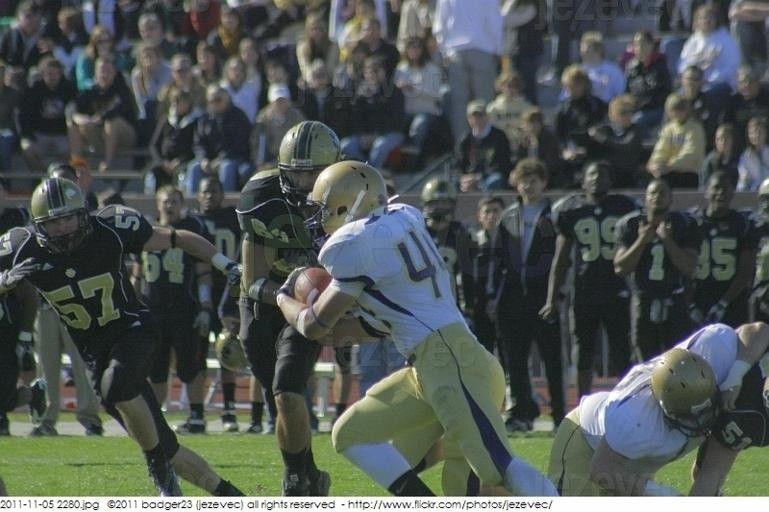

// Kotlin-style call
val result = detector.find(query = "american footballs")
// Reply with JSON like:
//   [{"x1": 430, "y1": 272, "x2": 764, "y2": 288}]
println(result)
[{"x1": 294, "y1": 267, "x2": 333, "y2": 306}]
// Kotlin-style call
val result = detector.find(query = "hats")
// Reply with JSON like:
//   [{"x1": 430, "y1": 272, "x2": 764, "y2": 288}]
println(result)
[
  {"x1": 267, "y1": 81, "x2": 291, "y2": 102},
  {"x1": 465, "y1": 97, "x2": 488, "y2": 119}
]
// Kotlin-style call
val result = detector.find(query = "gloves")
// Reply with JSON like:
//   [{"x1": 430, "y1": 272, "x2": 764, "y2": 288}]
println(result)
[
  {"x1": 273, "y1": 266, "x2": 310, "y2": 299},
  {"x1": 0, "y1": 254, "x2": 44, "y2": 301}
]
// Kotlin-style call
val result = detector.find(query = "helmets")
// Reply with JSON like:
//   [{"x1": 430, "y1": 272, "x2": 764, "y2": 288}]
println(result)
[
  {"x1": 419, "y1": 178, "x2": 458, "y2": 218},
  {"x1": 305, "y1": 158, "x2": 390, "y2": 237},
  {"x1": 650, "y1": 347, "x2": 723, "y2": 441},
  {"x1": 30, "y1": 176, "x2": 91, "y2": 254},
  {"x1": 276, "y1": 119, "x2": 341, "y2": 199}
]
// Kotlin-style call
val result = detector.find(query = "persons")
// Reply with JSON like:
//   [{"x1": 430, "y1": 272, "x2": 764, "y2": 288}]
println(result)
[{"x1": 0, "y1": 0, "x2": 768, "y2": 496}]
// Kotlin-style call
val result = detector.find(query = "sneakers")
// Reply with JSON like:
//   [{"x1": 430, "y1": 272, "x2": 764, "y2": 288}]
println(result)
[
  {"x1": 83, "y1": 424, "x2": 104, "y2": 435},
  {"x1": 282, "y1": 468, "x2": 332, "y2": 496},
  {"x1": 248, "y1": 423, "x2": 263, "y2": 434},
  {"x1": 27, "y1": 377, "x2": 49, "y2": 418},
  {"x1": 504, "y1": 415, "x2": 535, "y2": 434},
  {"x1": 223, "y1": 418, "x2": 238, "y2": 432},
  {"x1": 29, "y1": 421, "x2": 58, "y2": 437},
  {"x1": 264, "y1": 422, "x2": 275, "y2": 434},
  {"x1": 175, "y1": 417, "x2": 206, "y2": 434}
]
